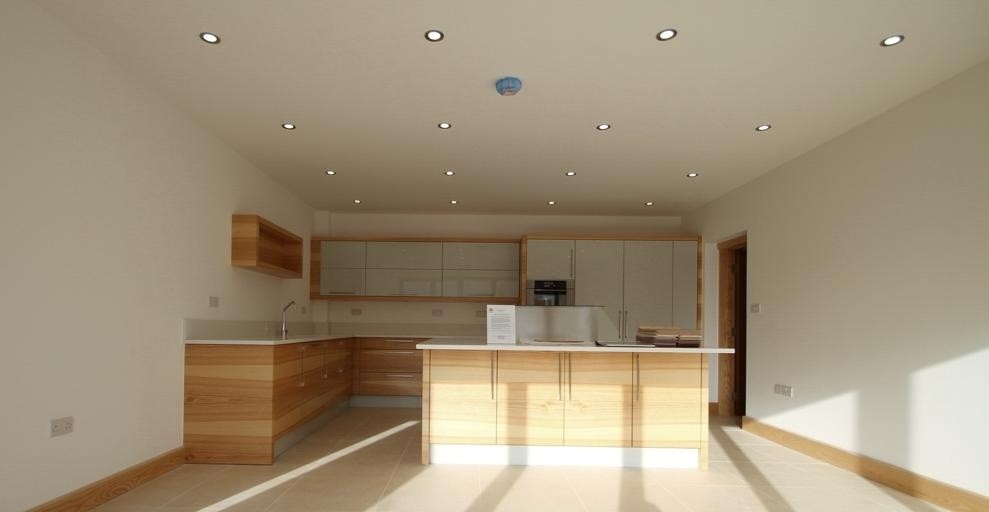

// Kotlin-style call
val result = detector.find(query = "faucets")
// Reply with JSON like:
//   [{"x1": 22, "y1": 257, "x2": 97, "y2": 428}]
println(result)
[{"x1": 281, "y1": 299, "x2": 297, "y2": 339}]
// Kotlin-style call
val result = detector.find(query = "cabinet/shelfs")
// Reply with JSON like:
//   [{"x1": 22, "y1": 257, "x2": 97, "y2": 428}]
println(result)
[
  {"x1": 354, "y1": 338, "x2": 424, "y2": 397},
  {"x1": 186, "y1": 339, "x2": 355, "y2": 465},
  {"x1": 528, "y1": 240, "x2": 574, "y2": 280},
  {"x1": 575, "y1": 242, "x2": 675, "y2": 343},
  {"x1": 311, "y1": 237, "x2": 520, "y2": 304},
  {"x1": 232, "y1": 214, "x2": 304, "y2": 275}
]
